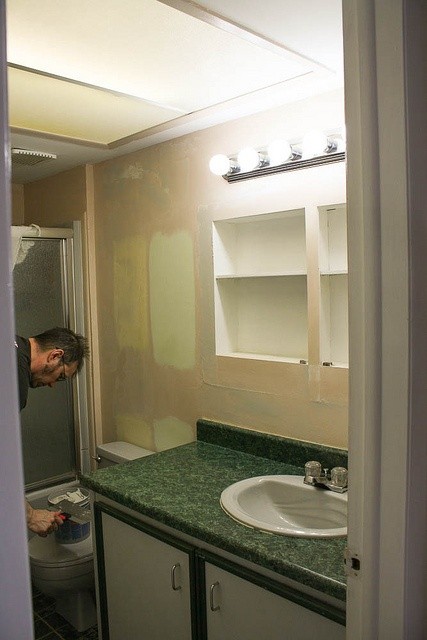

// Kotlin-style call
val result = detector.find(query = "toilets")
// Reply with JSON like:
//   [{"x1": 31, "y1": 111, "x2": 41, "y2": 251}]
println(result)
[{"x1": 29, "y1": 441, "x2": 158, "y2": 631}]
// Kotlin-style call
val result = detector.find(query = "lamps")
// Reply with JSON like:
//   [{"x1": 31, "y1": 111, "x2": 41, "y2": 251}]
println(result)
[{"x1": 209, "y1": 154, "x2": 240, "y2": 176}]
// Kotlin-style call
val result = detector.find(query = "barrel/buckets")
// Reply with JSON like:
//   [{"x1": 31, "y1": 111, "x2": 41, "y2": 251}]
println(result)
[{"x1": 48, "y1": 487, "x2": 91, "y2": 544}]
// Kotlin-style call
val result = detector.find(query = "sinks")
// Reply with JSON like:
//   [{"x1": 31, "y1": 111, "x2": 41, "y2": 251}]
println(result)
[{"x1": 234, "y1": 479, "x2": 348, "y2": 530}]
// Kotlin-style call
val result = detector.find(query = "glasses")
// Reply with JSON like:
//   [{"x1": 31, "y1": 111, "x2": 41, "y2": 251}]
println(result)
[{"x1": 56, "y1": 347, "x2": 65, "y2": 381}]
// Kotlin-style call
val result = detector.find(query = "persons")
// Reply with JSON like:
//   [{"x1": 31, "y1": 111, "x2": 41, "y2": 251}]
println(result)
[{"x1": 15, "y1": 327, "x2": 89, "y2": 538}]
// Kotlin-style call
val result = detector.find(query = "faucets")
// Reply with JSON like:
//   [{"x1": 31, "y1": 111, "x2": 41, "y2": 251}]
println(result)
[{"x1": 303, "y1": 474, "x2": 329, "y2": 485}]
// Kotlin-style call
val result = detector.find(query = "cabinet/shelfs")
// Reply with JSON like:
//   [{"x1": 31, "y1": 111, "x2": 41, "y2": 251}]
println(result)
[
  {"x1": 79, "y1": 471, "x2": 346, "y2": 639},
  {"x1": 199, "y1": 138, "x2": 350, "y2": 407}
]
